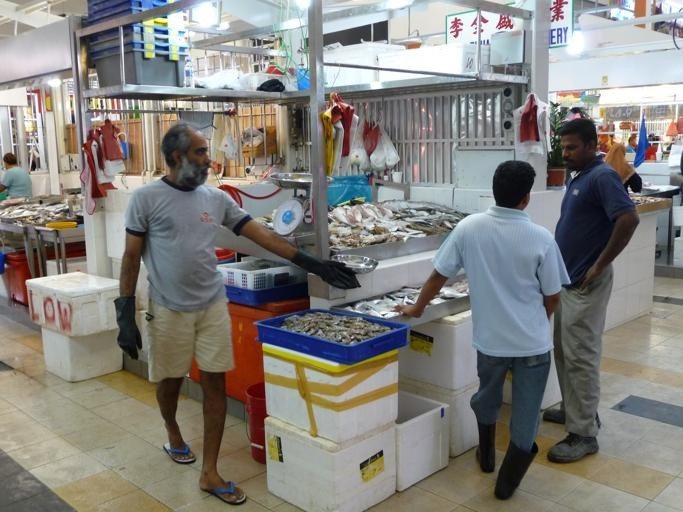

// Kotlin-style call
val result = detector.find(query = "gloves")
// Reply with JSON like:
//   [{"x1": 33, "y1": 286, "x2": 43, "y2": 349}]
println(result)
[
  {"x1": 293, "y1": 248, "x2": 361, "y2": 289},
  {"x1": 114, "y1": 296, "x2": 142, "y2": 359}
]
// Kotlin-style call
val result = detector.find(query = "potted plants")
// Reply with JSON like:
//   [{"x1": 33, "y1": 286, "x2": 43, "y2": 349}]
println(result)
[{"x1": 547, "y1": 101, "x2": 569, "y2": 186}]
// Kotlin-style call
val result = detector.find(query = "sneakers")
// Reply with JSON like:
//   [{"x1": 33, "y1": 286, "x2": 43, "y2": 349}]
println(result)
[
  {"x1": 544, "y1": 409, "x2": 600, "y2": 429},
  {"x1": 548, "y1": 432, "x2": 599, "y2": 463}
]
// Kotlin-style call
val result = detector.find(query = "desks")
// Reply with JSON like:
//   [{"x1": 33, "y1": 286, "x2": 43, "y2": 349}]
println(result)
[{"x1": 625, "y1": 185, "x2": 680, "y2": 265}]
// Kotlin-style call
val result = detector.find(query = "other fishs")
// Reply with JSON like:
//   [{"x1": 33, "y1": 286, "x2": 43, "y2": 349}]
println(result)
[
  {"x1": 327, "y1": 199, "x2": 469, "y2": 252},
  {"x1": 342, "y1": 285, "x2": 469, "y2": 319},
  {"x1": 0, "y1": 202, "x2": 69, "y2": 227}
]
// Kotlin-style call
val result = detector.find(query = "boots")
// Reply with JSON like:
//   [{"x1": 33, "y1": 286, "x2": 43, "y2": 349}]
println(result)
[
  {"x1": 475, "y1": 421, "x2": 496, "y2": 471},
  {"x1": 495, "y1": 441, "x2": 538, "y2": 500}
]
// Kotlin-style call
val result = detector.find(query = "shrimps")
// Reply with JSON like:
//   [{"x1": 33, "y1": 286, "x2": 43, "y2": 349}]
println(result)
[{"x1": 279, "y1": 311, "x2": 395, "y2": 345}]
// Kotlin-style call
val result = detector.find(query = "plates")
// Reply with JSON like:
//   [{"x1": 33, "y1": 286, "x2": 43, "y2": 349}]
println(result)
[{"x1": 45, "y1": 222, "x2": 76, "y2": 228}]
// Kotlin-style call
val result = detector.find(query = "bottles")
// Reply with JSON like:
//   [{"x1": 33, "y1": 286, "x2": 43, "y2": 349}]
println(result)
[{"x1": 608, "y1": 119, "x2": 614, "y2": 132}]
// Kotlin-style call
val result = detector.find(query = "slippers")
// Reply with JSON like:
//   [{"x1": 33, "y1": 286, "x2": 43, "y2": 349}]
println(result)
[
  {"x1": 200, "y1": 477, "x2": 246, "y2": 505},
  {"x1": 164, "y1": 442, "x2": 196, "y2": 463}
]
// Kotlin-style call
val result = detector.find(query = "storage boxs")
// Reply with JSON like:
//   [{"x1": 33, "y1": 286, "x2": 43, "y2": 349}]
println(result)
[
  {"x1": 190, "y1": 296, "x2": 566, "y2": 512},
  {"x1": 296, "y1": 41, "x2": 490, "y2": 91},
  {"x1": 82, "y1": 0, "x2": 191, "y2": 87},
  {"x1": 5, "y1": 189, "x2": 149, "y2": 382}
]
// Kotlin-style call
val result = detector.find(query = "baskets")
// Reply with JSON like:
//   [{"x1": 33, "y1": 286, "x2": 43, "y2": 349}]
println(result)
[{"x1": 216, "y1": 261, "x2": 306, "y2": 290}]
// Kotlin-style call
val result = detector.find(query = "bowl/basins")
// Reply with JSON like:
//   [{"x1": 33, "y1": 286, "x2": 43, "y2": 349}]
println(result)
[
  {"x1": 329, "y1": 254, "x2": 378, "y2": 273},
  {"x1": 213, "y1": 249, "x2": 236, "y2": 265}
]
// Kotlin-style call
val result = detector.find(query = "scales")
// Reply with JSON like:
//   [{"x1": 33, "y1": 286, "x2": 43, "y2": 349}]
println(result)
[{"x1": 269, "y1": 172, "x2": 333, "y2": 245}]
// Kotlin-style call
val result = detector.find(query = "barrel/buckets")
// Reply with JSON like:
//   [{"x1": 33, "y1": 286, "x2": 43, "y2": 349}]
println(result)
[{"x1": 242, "y1": 381, "x2": 269, "y2": 467}]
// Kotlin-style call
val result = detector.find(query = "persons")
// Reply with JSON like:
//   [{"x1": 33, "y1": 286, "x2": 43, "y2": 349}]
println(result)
[
  {"x1": 393, "y1": 159, "x2": 572, "y2": 501},
  {"x1": 543, "y1": 120, "x2": 641, "y2": 464},
  {"x1": 623, "y1": 137, "x2": 637, "y2": 153},
  {"x1": 0, "y1": 152, "x2": 33, "y2": 201},
  {"x1": 111, "y1": 122, "x2": 361, "y2": 505}
]
